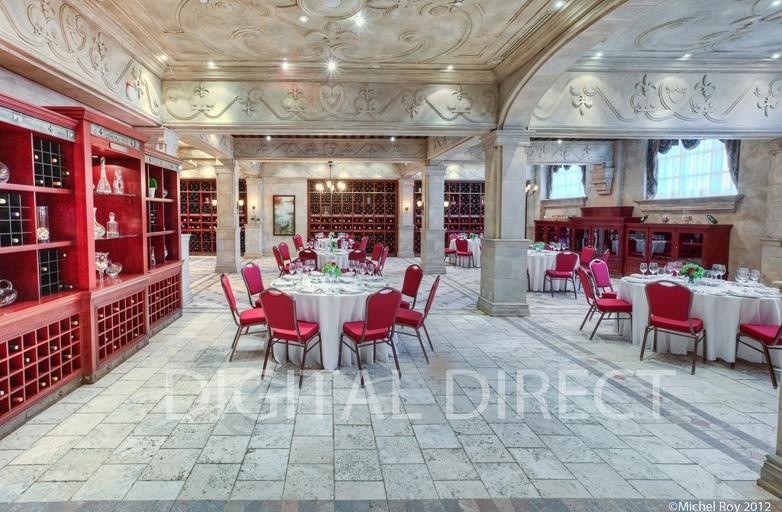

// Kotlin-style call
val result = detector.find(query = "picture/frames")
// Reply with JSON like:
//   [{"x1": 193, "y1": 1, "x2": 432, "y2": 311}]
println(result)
[{"x1": 273, "y1": 194, "x2": 296, "y2": 237}]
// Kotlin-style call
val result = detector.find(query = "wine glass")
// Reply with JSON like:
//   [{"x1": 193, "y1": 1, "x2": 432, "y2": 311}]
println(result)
[
  {"x1": 734, "y1": 268, "x2": 760, "y2": 289},
  {"x1": 711, "y1": 264, "x2": 719, "y2": 285},
  {"x1": 649, "y1": 263, "x2": 659, "y2": 280},
  {"x1": 658, "y1": 266, "x2": 666, "y2": 282},
  {"x1": 562, "y1": 243, "x2": 567, "y2": 254},
  {"x1": 305, "y1": 259, "x2": 316, "y2": 279},
  {"x1": 295, "y1": 261, "x2": 303, "y2": 276},
  {"x1": 664, "y1": 262, "x2": 681, "y2": 280},
  {"x1": 549, "y1": 240, "x2": 562, "y2": 254},
  {"x1": 348, "y1": 260, "x2": 375, "y2": 283},
  {"x1": 639, "y1": 262, "x2": 648, "y2": 281},
  {"x1": 288, "y1": 262, "x2": 297, "y2": 280},
  {"x1": 702, "y1": 269, "x2": 717, "y2": 292},
  {"x1": 717, "y1": 264, "x2": 726, "y2": 285}
]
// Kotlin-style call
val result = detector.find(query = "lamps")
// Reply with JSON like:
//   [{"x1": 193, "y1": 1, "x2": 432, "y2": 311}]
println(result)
[
  {"x1": 525, "y1": 184, "x2": 537, "y2": 239},
  {"x1": 315, "y1": 161, "x2": 345, "y2": 194}
]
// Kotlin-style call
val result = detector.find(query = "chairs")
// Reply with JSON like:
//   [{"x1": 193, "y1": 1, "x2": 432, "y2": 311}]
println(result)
[
  {"x1": 543, "y1": 247, "x2": 782, "y2": 390},
  {"x1": 444, "y1": 234, "x2": 474, "y2": 269},
  {"x1": 219, "y1": 233, "x2": 440, "y2": 389}
]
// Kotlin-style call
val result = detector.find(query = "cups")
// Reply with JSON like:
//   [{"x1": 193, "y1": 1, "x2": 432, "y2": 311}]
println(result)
[
  {"x1": 36, "y1": 205, "x2": 50, "y2": 244},
  {"x1": 95, "y1": 252, "x2": 110, "y2": 283}
]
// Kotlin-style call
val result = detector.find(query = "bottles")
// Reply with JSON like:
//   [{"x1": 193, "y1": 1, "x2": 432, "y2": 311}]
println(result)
[
  {"x1": 9, "y1": 342, "x2": 19, "y2": 356},
  {"x1": 94, "y1": 155, "x2": 111, "y2": 195},
  {"x1": 61, "y1": 350, "x2": 71, "y2": 362},
  {"x1": 706, "y1": 214, "x2": 718, "y2": 224},
  {"x1": 93, "y1": 207, "x2": 106, "y2": 239},
  {"x1": 640, "y1": 214, "x2": 650, "y2": 223},
  {"x1": 150, "y1": 245, "x2": 156, "y2": 268},
  {"x1": 112, "y1": 168, "x2": 125, "y2": 195},
  {"x1": 58, "y1": 282, "x2": 75, "y2": 291},
  {"x1": 106, "y1": 211, "x2": 119, "y2": 236}
]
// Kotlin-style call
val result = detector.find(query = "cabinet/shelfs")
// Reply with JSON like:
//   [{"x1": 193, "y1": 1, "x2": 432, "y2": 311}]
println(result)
[
  {"x1": 624, "y1": 222, "x2": 734, "y2": 280},
  {"x1": 570, "y1": 205, "x2": 645, "y2": 276},
  {"x1": 0, "y1": 93, "x2": 185, "y2": 440},
  {"x1": 534, "y1": 219, "x2": 571, "y2": 250}
]
